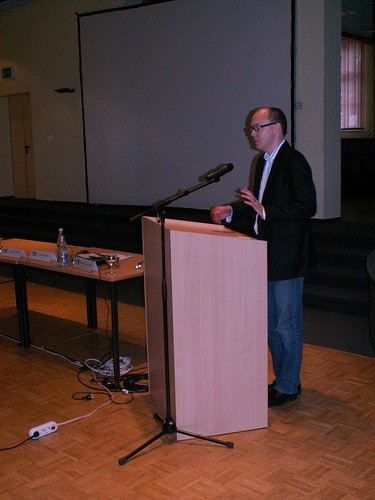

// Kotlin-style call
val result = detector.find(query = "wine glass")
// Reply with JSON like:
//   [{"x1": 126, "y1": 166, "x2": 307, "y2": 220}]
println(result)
[{"x1": 106, "y1": 254, "x2": 118, "y2": 275}]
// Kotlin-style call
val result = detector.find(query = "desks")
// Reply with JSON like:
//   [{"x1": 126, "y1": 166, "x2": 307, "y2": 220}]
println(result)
[{"x1": 0, "y1": 238, "x2": 145, "y2": 386}]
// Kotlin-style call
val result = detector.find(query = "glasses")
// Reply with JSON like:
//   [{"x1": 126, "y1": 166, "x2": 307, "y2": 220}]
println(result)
[{"x1": 250, "y1": 123, "x2": 276, "y2": 131}]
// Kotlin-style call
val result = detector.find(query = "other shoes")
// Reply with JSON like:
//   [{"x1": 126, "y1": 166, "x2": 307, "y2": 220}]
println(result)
[
  {"x1": 268, "y1": 387, "x2": 298, "y2": 407},
  {"x1": 268, "y1": 380, "x2": 301, "y2": 395}
]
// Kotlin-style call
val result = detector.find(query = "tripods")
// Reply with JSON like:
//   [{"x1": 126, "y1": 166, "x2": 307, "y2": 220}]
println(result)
[{"x1": 118, "y1": 178, "x2": 234, "y2": 465}]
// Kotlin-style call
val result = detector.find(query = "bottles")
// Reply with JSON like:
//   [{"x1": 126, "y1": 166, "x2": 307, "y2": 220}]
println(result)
[{"x1": 56, "y1": 228, "x2": 68, "y2": 266}]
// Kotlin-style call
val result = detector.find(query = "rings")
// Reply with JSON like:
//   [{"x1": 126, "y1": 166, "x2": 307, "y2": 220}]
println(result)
[{"x1": 253, "y1": 199, "x2": 256, "y2": 203}]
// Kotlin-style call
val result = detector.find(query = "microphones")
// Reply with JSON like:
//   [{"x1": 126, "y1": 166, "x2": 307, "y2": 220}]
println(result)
[{"x1": 206, "y1": 164, "x2": 234, "y2": 181}]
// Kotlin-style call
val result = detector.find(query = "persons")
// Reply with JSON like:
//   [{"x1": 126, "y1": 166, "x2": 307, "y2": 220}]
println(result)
[{"x1": 210, "y1": 106, "x2": 317, "y2": 407}]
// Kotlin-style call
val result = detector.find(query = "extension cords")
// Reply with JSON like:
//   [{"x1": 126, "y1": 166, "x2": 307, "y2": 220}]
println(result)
[{"x1": 29, "y1": 421, "x2": 58, "y2": 439}]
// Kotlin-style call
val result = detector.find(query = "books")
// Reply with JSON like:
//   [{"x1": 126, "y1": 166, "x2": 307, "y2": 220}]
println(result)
[{"x1": 72, "y1": 254, "x2": 118, "y2": 272}]
